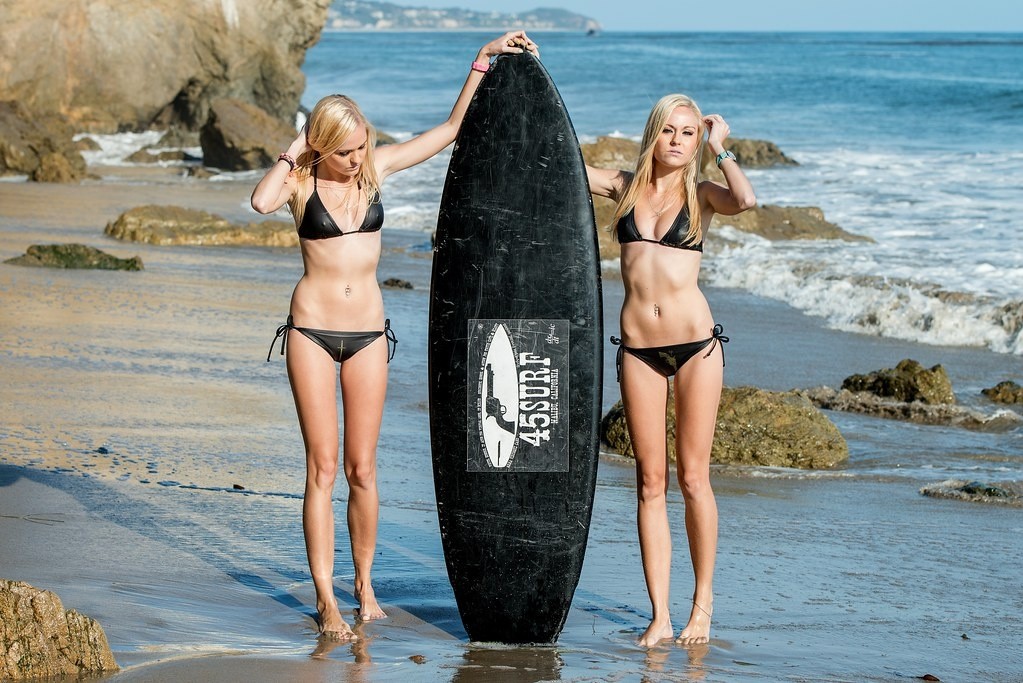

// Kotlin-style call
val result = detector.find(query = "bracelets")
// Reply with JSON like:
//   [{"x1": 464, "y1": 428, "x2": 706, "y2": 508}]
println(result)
[
  {"x1": 278, "y1": 158, "x2": 293, "y2": 171},
  {"x1": 280, "y1": 153, "x2": 296, "y2": 167},
  {"x1": 472, "y1": 62, "x2": 489, "y2": 72}
]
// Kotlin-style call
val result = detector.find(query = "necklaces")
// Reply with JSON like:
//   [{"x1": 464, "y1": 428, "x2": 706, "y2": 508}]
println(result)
[
  {"x1": 326, "y1": 183, "x2": 350, "y2": 212},
  {"x1": 648, "y1": 196, "x2": 664, "y2": 217}
]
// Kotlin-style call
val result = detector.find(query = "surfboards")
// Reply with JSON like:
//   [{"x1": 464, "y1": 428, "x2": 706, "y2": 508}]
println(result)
[{"x1": 427, "y1": 50, "x2": 603, "y2": 645}]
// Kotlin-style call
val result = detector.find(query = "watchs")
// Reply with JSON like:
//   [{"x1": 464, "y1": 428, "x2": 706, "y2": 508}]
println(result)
[{"x1": 717, "y1": 151, "x2": 736, "y2": 168}]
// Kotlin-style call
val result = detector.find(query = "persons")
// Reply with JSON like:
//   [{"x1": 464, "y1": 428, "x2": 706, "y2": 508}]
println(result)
[
  {"x1": 507, "y1": 37, "x2": 756, "y2": 647},
  {"x1": 251, "y1": 30, "x2": 540, "y2": 639}
]
